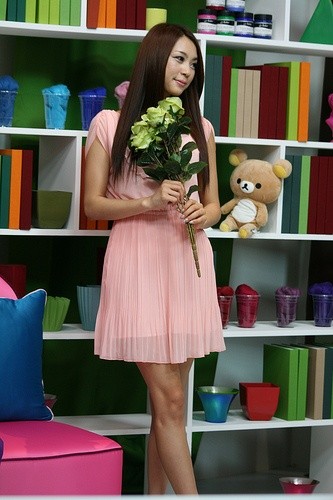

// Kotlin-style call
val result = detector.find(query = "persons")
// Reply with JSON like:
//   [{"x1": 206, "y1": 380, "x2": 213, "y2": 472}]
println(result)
[{"x1": 84, "y1": 23, "x2": 226, "y2": 495}]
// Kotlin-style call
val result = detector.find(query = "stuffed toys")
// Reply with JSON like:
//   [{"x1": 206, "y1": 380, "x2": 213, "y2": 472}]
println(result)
[{"x1": 219, "y1": 149, "x2": 292, "y2": 238}]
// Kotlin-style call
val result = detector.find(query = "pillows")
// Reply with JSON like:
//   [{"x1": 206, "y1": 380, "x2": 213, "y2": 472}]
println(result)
[{"x1": 0, "y1": 290, "x2": 56, "y2": 423}]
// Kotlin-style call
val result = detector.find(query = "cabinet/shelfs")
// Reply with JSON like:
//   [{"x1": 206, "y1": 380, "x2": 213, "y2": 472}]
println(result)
[{"x1": 0, "y1": 0, "x2": 333, "y2": 495}]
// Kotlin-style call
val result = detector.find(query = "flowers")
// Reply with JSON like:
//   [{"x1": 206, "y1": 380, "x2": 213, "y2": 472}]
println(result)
[{"x1": 127, "y1": 95, "x2": 201, "y2": 277}]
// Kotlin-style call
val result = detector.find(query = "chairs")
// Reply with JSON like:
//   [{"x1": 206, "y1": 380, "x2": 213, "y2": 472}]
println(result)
[{"x1": 0, "y1": 275, "x2": 123, "y2": 495}]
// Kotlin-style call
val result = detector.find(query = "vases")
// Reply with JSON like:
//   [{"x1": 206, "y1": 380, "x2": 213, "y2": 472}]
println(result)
[
  {"x1": 42, "y1": 296, "x2": 70, "y2": 332},
  {"x1": 31, "y1": 189, "x2": 73, "y2": 229},
  {"x1": 76, "y1": 285, "x2": 102, "y2": 332},
  {"x1": 238, "y1": 381, "x2": 281, "y2": 420}
]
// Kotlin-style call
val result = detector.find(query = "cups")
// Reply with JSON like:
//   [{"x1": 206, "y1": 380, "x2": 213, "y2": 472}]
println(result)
[
  {"x1": 77, "y1": 95, "x2": 107, "y2": 131},
  {"x1": 195, "y1": 385, "x2": 239, "y2": 423},
  {"x1": 278, "y1": 476, "x2": 319, "y2": 495},
  {"x1": 38, "y1": 296, "x2": 72, "y2": 332},
  {"x1": 31, "y1": 189, "x2": 73, "y2": 228},
  {"x1": 0, "y1": 89, "x2": 18, "y2": 127},
  {"x1": 308, "y1": 294, "x2": 333, "y2": 328},
  {"x1": 42, "y1": 91, "x2": 71, "y2": 130},
  {"x1": 236, "y1": 294, "x2": 262, "y2": 328},
  {"x1": 275, "y1": 295, "x2": 300, "y2": 328},
  {"x1": 76, "y1": 285, "x2": 103, "y2": 330},
  {"x1": 215, "y1": 294, "x2": 235, "y2": 330}
]
